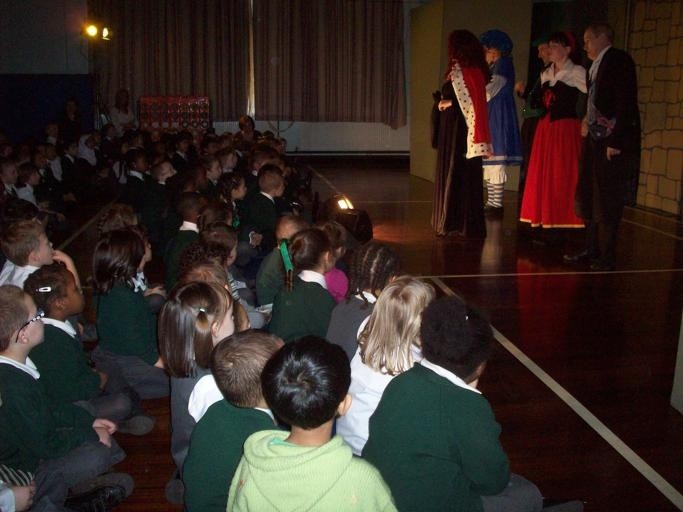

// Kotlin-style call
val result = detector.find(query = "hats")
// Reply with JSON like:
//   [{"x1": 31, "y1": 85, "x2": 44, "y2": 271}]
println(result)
[{"x1": 447, "y1": 30, "x2": 576, "y2": 54}]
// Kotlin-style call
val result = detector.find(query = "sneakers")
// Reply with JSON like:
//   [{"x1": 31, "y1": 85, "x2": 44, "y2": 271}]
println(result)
[{"x1": 114, "y1": 408, "x2": 154, "y2": 436}]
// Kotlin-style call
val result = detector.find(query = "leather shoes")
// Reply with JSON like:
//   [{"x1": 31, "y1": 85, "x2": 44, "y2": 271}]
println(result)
[
  {"x1": 482, "y1": 204, "x2": 615, "y2": 272},
  {"x1": 63, "y1": 470, "x2": 135, "y2": 511}
]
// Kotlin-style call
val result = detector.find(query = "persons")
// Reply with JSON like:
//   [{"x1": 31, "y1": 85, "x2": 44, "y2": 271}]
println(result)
[
  {"x1": 559, "y1": 20, "x2": 644, "y2": 275},
  {"x1": 509, "y1": 32, "x2": 553, "y2": 236},
  {"x1": 358, "y1": 291, "x2": 548, "y2": 511},
  {"x1": 473, "y1": 29, "x2": 530, "y2": 216},
  {"x1": 1, "y1": 89, "x2": 445, "y2": 512},
  {"x1": 430, "y1": 29, "x2": 495, "y2": 241},
  {"x1": 508, "y1": 30, "x2": 587, "y2": 249}
]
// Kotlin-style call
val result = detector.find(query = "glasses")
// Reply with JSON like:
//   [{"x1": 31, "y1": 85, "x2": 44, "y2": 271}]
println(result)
[{"x1": 15, "y1": 306, "x2": 46, "y2": 343}]
